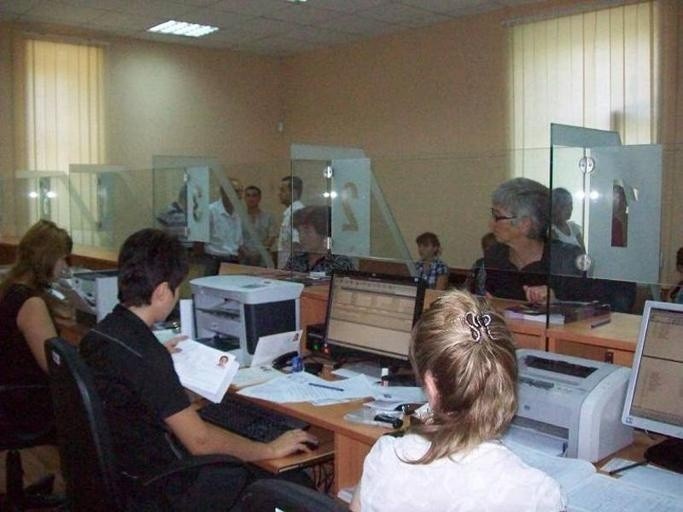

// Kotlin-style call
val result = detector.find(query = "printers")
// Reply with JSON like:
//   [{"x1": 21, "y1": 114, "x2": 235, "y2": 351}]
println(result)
[
  {"x1": 501, "y1": 348, "x2": 633, "y2": 463},
  {"x1": 72, "y1": 269, "x2": 120, "y2": 334},
  {"x1": 189, "y1": 275, "x2": 305, "y2": 365}
]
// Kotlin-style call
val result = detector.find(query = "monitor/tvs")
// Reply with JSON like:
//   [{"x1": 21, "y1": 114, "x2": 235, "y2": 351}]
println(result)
[
  {"x1": 322, "y1": 269, "x2": 426, "y2": 368},
  {"x1": 621, "y1": 300, "x2": 683, "y2": 474}
]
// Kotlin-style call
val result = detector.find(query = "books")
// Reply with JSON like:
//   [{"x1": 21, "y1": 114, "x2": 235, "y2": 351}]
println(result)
[
  {"x1": 500, "y1": 438, "x2": 682, "y2": 511},
  {"x1": 169, "y1": 335, "x2": 240, "y2": 404}
]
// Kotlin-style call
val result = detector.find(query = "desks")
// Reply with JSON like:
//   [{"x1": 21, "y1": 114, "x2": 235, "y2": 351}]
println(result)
[{"x1": 1, "y1": 234, "x2": 683, "y2": 497}]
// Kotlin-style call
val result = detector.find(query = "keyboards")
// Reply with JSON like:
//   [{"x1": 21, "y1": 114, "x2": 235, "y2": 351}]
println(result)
[{"x1": 199, "y1": 396, "x2": 310, "y2": 444}]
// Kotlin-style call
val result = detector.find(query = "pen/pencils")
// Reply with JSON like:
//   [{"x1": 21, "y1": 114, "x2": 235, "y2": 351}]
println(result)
[
  {"x1": 309, "y1": 383, "x2": 343, "y2": 391},
  {"x1": 591, "y1": 319, "x2": 612, "y2": 328},
  {"x1": 609, "y1": 460, "x2": 647, "y2": 475}
]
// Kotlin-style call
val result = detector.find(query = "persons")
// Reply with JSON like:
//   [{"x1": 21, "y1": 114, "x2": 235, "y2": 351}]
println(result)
[
  {"x1": 484, "y1": 177, "x2": 583, "y2": 306},
  {"x1": 346, "y1": 286, "x2": 565, "y2": 512},
  {"x1": 461, "y1": 232, "x2": 498, "y2": 297},
  {"x1": 0, "y1": 218, "x2": 73, "y2": 432},
  {"x1": 665, "y1": 247, "x2": 682, "y2": 304},
  {"x1": 79, "y1": 227, "x2": 318, "y2": 512},
  {"x1": 281, "y1": 205, "x2": 356, "y2": 276},
  {"x1": 276, "y1": 176, "x2": 304, "y2": 271},
  {"x1": 193, "y1": 178, "x2": 243, "y2": 277},
  {"x1": 238, "y1": 186, "x2": 278, "y2": 270},
  {"x1": 214, "y1": 356, "x2": 228, "y2": 369},
  {"x1": 155, "y1": 184, "x2": 187, "y2": 241},
  {"x1": 611, "y1": 185, "x2": 627, "y2": 247},
  {"x1": 544, "y1": 187, "x2": 583, "y2": 249},
  {"x1": 413, "y1": 232, "x2": 449, "y2": 291}
]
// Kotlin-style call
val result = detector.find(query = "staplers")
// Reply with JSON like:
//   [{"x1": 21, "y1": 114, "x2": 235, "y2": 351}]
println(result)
[{"x1": 271, "y1": 350, "x2": 298, "y2": 370}]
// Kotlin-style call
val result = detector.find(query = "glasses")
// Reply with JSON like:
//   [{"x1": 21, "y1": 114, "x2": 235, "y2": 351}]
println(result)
[{"x1": 490, "y1": 207, "x2": 517, "y2": 221}]
